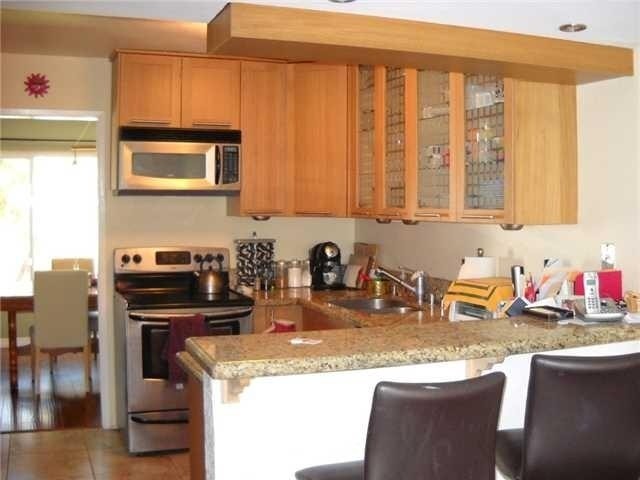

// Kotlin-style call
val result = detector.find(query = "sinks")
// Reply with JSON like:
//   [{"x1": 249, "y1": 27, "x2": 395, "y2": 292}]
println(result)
[
  {"x1": 328, "y1": 299, "x2": 407, "y2": 308},
  {"x1": 358, "y1": 307, "x2": 419, "y2": 315}
]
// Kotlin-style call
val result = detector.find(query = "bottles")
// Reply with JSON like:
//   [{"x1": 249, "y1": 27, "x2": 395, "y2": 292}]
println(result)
[{"x1": 261, "y1": 260, "x2": 312, "y2": 290}]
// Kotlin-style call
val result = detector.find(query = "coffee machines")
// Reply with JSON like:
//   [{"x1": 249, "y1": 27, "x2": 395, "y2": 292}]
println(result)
[{"x1": 310, "y1": 242, "x2": 347, "y2": 291}]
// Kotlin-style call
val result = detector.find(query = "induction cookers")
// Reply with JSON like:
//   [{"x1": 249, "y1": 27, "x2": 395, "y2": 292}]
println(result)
[{"x1": 115, "y1": 288, "x2": 256, "y2": 311}]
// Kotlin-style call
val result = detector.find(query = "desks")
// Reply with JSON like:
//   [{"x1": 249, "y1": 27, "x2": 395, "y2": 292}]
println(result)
[{"x1": 0, "y1": 286, "x2": 98, "y2": 398}]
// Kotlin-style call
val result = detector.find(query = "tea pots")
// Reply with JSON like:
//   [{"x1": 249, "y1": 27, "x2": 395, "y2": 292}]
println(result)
[{"x1": 194, "y1": 257, "x2": 227, "y2": 294}]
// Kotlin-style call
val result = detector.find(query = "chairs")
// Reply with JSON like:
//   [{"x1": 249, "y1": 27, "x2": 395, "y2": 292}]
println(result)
[
  {"x1": 497, "y1": 353, "x2": 640, "y2": 480},
  {"x1": 294, "y1": 359, "x2": 506, "y2": 480},
  {"x1": 28, "y1": 257, "x2": 95, "y2": 399}
]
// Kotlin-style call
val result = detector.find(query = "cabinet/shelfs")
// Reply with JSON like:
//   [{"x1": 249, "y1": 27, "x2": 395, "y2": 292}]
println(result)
[
  {"x1": 501, "y1": 59, "x2": 578, "y2": 224},
  {"x1": 240, "y1": 51, "x2": 349, "y2": 219},
  {"x1": 413, "y1": 62, "x2": 514, "y2": 224},
  {"x1": 349, "y1": 55, "x2": 416, "y2": 223},
  {"x1": 112, "y1": 48, "x2": 241, "y2": 135}
]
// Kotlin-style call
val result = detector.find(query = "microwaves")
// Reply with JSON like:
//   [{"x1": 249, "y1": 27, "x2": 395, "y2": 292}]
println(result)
[{"x1": 116, "y1": 139, "x2": 242, "y2": 195}]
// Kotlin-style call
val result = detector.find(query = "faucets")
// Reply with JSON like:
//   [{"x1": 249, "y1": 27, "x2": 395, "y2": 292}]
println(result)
[{"x1": 375, "y1": 266, "x2": 425, "y2": 305}]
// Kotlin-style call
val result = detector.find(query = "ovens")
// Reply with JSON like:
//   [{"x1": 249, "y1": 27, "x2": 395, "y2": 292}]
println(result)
[{"x1": 125, "y1": 310, "x2": 253, "y2": 456}]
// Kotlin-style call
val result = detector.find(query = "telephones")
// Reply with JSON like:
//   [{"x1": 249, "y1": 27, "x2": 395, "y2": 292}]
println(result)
[{"x1": 573, "y1": 271, "x2": 624, "y2": 321}]
